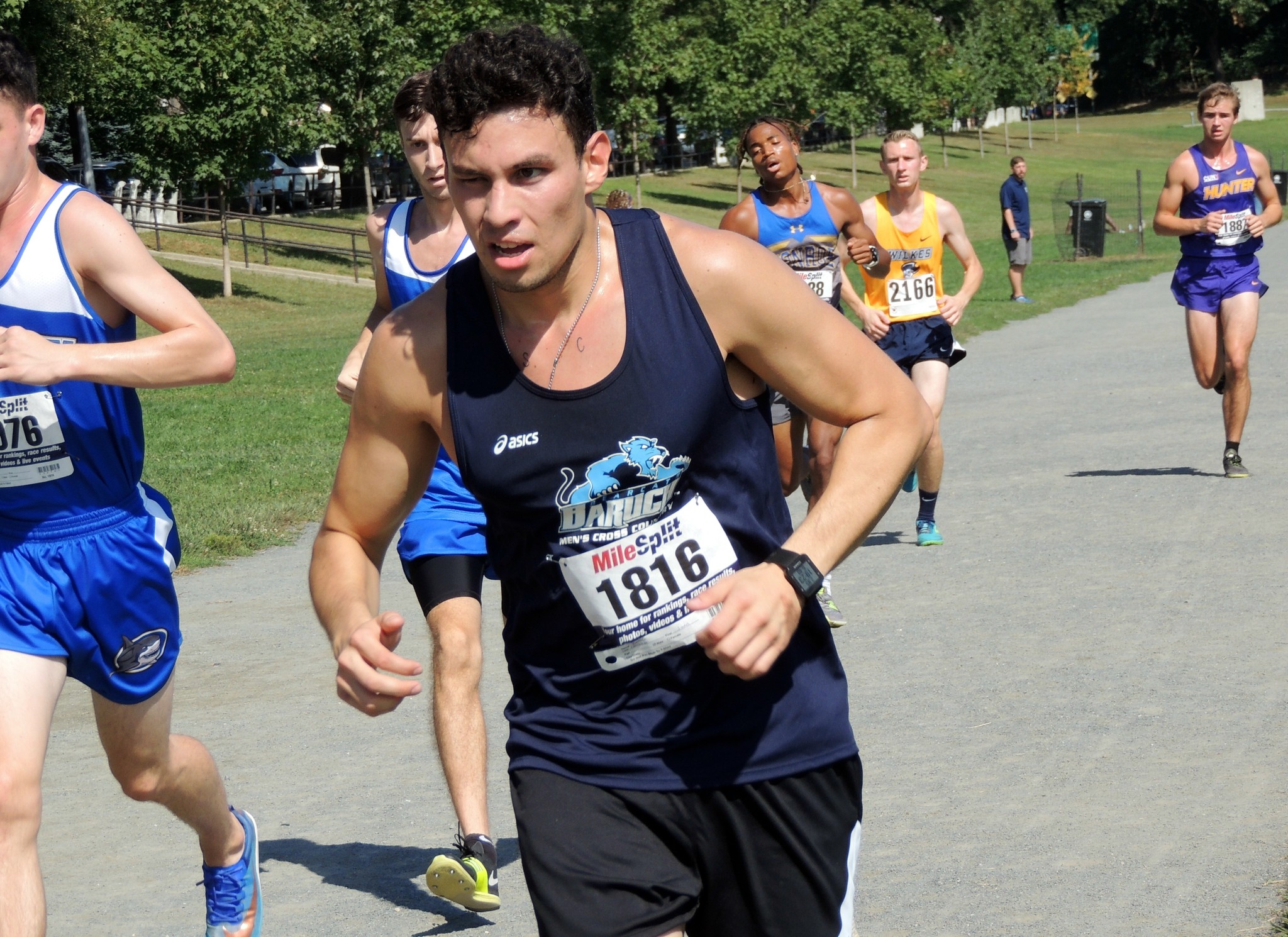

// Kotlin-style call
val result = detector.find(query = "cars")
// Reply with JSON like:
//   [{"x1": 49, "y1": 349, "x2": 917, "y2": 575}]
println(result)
[
  {"x1": 1025, "y1": 95, "x2": 1076, "y2": 119},
  {"x1": 803, "y1": 109, "x2": 840, "y2": 143},
  {"x1": 179, "y1": 143, "x2": 347, "y2": 221},
  {"x1": 603, "y1": 115, "x2": 749, "y2": 170}
]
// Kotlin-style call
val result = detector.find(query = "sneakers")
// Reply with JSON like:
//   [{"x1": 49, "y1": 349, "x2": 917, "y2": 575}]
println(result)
[
  {"x1": 1222, "y1": 448, "x2": 1249, "y2": 477},
  {"x1": 1214, "y1": 340, "x2": 1226, "y2": 394},
  {"x1": 425, "y1": 821, "x2": 502, "y2": 913},
  {"x1": 196, "y1": 804, "x2": 262, "y2": 936},
  {"x1": 916, "y1": 515, "x2": 944, "y2": 546},
  {"x1": 901, "y1": 467, "x2": 917, "y2": 493},
  {"x1": 816, "y1": 586, "x2": 847, "y2": 628}
]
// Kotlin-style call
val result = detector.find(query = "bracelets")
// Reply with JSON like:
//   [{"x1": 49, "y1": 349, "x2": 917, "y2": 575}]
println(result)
[{"x1": 1010, "y1": 228, "x2": 1017, "y2": 233}]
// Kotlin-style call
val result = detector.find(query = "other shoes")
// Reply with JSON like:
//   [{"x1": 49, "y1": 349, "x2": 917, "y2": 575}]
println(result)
[{"x1": 1010, "y1": 294, "x2": 1032, "y2": 303}]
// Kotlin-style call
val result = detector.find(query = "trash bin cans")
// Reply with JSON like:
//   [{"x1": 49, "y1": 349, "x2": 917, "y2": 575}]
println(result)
[
  {"x1": 1271, "y1": 169, "x2": 1288, "y2": 207},
  {"x1": 1066, "y1": 200, "x2": 1107, "y2": 258}
]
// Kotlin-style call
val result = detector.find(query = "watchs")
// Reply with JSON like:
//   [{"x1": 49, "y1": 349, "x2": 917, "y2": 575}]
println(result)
[
  {"x1": 862, "y1": 245, "x2": 880, "y2": 270},
  {"x1": 764, "y1": 548, "x2": 824, "y2": 600}
]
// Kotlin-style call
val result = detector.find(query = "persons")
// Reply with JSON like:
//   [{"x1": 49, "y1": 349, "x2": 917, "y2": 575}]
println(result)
[
  {"x1": 0, "y1": 34, "x2": 264, "y2": 936},
  {"x1": 827, "y1": 130, "x2": 983, "y2": 547},
  {"x1": 307, "y1": 21, "x2": 933, "y2": 936},
  {"x1": 337, "y1": 76, "x2": 529, "y2": 913},
  {"x1": 721, "y1": 116, "x2": 892, "y2": 621},
  {"x1": 1153, "y1": 81, "x2": 1282, "y2": 477},
  {"x1": 999, "y1": 156, "x2": 1034, "y2": 302}
]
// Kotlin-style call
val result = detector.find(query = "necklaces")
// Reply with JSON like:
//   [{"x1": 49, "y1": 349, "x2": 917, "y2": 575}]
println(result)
[
  {"x1": 491, "y1": 220, "x2": 602, "y2": 390},
  {"x1": 763, "y1": 174, "x2": 816, "y2": 202}
]
